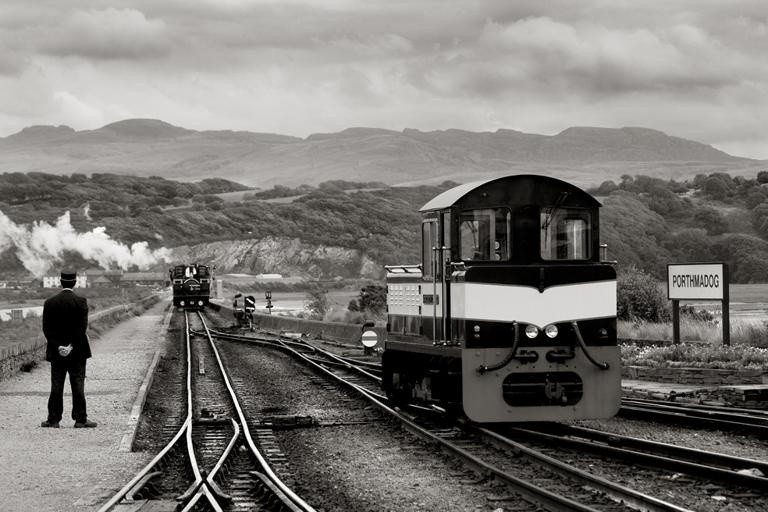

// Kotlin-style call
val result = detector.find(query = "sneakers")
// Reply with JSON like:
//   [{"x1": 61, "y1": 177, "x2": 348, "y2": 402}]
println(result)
[
  {"x1": 74, "y1": 420, "x2": 97, "y2": 428},
  {"x1": 41, "y1": 421, "x2": 59, "y2": 428}
]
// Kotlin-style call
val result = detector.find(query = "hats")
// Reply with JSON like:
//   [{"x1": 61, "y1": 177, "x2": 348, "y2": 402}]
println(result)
[{"x1": 60, "y1": 269, "x2": 76, "y2": 282}]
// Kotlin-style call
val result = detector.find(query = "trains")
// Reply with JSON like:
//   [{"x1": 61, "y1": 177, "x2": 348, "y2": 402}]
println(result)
[
  {"x1": 380, "y1": 174, "x2": 625, "y2": 426},
  {"x1": 169, "y1": 263, "x2": 210, "y2": 311}
]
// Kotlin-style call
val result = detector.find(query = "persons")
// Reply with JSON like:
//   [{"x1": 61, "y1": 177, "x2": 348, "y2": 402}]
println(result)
[{"x1": 37, "y1": 268, "x2": 97, "y2": 428}]
